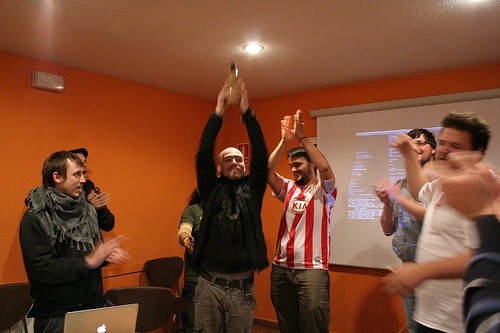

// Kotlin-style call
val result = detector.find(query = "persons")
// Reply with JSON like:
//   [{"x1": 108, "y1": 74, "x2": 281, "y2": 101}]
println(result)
[
  {"x1": 178, "y1": 184, "x2": 204, "y2": 333},
  {"x1": 266, "y1": 109, "x2": 337, "y2": 333},
  {"x1": 375, "y1": 128, "x2": 436, "y2": 333},
  {"x1": 193, "y1": 76, "x2": 270, "y2": 333},
  {"x1": 381, "y1": 113, "x2": 492, "y2": 333},
  {"x1": 19, "y1": 149, "x2": 130, "y2": 333},
  {"x1": 429, "y1": 153, "x2": 500, "y2": 333},
  {"x1": 68, "y1": 148, "x2": 115, "y2": 232}
]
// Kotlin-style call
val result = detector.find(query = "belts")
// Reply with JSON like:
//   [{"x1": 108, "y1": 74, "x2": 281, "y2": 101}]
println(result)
[{"x1": 198, "y1": 268, "x2": 257, "y2": 310}]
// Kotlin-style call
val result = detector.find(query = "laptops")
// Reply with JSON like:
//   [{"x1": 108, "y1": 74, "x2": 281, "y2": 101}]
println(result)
[{"x1": 63, "y1": 303, "x2": 139, "y2": 333}]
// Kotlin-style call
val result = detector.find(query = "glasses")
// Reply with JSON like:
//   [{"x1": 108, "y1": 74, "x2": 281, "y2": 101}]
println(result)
[{"x1": 414, "y1": 139, "x2": 433, "y2": 148}]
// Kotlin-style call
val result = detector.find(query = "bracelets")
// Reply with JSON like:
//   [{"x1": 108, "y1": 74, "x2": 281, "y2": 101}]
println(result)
[
  {"x1": 183, "y1": 237, "x2": 189, "y2": 246},
  {"x1": 299, "y1": 136, "x2": 307, "y2": 142}
]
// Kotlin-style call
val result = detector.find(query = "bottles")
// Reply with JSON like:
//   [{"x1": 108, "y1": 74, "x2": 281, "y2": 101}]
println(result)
[{"x1": 227, "y1": 60, "x2": 241, "y2": 104}]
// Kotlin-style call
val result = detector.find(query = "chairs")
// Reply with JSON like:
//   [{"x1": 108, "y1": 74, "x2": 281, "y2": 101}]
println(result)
[{"x1": 0, "y1": 256, "x2": 185, "y2": 333}]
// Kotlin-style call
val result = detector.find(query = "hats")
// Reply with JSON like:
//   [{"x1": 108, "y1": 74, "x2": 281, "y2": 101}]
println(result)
[{"x1": 69, "y1": 147, "x2": 88, "y2": 159}]
